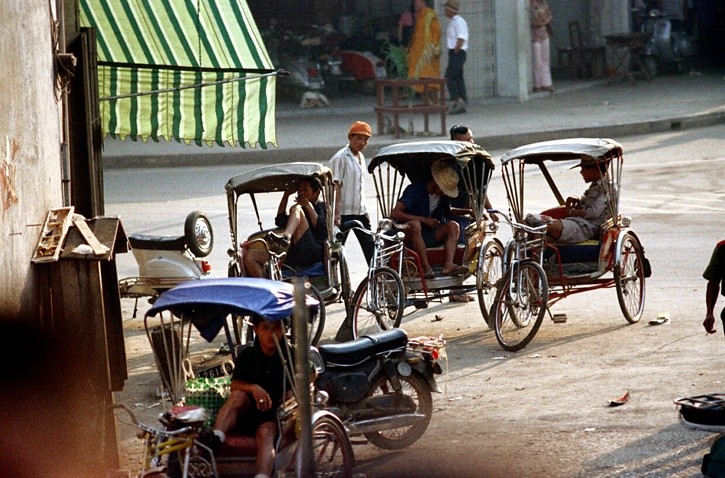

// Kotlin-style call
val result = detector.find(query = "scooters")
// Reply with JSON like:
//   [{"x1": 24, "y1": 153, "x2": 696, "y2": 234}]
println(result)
[
  {"x1": 115, "y1": 210, "x2": 218, "y2": 320},
  {"x1": 258, "y1": 24, "x2": 329, "y2": 94},
  {"x1": 631, "y1": 5, "x2": 697, "y2": 80},
  {"x1": 304, "y1": 23, "x2": 342, "y2": 95}
]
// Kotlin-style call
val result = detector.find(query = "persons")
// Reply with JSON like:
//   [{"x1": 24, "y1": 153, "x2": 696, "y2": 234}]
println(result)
[
  {"x1": 390, "y1": 159, "x2": 469, "y2": 278},
  {"x1": 702, "y1": 239, "x2": 725, "y2": 344},
  {"x1": 197, "y1": 311, "x2": 318, "y2": 478},
  {"x1": 326, "y1": 121, "x2": 377, "y2": 268},
  {"x1": 524, "y1": 153, "x2": 617, "y2": 259},
  {"x1": 529, "y1": 0, "x2": 556, "y2": 93},
  {"x1": 395, "y1": 0, "x2": 443, "y2": 102},
  {"x1": 264, "y1": 176, "x2": 329, "y2": 272},
  {"x1": 447, "y1": 124, "x2": 500, "y2": 304},
  {"x1": 439, "y1": 0, "x2": 469, "y2": 115}
]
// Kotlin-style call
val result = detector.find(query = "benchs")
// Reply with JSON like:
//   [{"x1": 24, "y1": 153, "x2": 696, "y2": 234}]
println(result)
[
  {"x1": 539, "y1": 205, "x2": 603, "y2": 261},
  {"x1": 249, "y1": 228, "x2": 325, "y2": 277},
  {"x1": 422, "y1": 211, "x2": 477, "y2": 260},
  {"x1": 184, "y1": 378, "x2": 283, "y2": 456}
]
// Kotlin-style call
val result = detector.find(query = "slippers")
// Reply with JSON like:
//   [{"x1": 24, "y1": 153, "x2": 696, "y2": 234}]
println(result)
[
  {"x1": 441, "y1": 264, "x2": 469, "y2": 275},
  {"x1": 425, "y1": 268, "x2": 435, "y2": 278}
]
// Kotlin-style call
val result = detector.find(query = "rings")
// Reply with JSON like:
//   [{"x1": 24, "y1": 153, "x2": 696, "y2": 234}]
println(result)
[{"x1": 259, "y1": 399, "x2": 263, "y2": 403}]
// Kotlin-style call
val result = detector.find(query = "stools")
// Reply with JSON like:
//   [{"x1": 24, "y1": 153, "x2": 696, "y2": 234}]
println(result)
[{"x1": 557, "y1": 47, "x2": 588, "y2": 84}]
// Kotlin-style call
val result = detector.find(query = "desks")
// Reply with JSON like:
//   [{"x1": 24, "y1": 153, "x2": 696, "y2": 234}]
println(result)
[{"x1": 604, "y1": 32, "x2": 653, "y2": 87}]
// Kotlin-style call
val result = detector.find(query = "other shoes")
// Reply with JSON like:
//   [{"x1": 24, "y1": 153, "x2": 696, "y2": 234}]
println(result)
[
  {"x1": 268, "y1": 231, "x2": 290, "y2": 250},
  {"x1": 449, "y1": 292, "x2": 469, "y2": 303},
  {"x1": 449, "y1": 99, "x2": 466, "y2": 115},
  {"x1": 199, "y1": 428, "x2": 223, "y2": 458}
]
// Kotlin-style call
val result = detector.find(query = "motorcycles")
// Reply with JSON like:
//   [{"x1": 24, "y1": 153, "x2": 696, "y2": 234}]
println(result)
[{"x1": 312, "y1": 328, "x2": 446, "y2": 449}]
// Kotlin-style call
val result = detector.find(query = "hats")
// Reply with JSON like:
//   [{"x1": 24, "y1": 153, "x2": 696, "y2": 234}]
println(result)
[
  {"x1": 431, "y1": 159, "x2": 459, "y2": 198},
  {"x1": 349, "y1": 121, "x2": 372, "y2": 137},
  {"x1": 569, "y1": 154, "x2": 613, "y2": 170},
  {"x1": 441, "y1": 0, "x2": 461, "y2": 15}
]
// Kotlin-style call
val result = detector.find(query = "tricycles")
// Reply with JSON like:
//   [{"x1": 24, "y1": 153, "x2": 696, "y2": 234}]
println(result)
[
  {"x1": 491, "y1": 138, "x2": 653, "y2": 351},
  {"x1": 348, "y1": 142, "x2": 507, "y2": 347},
  {"x1": 110, "y1": 275, "x2": 321, "y2": 478},
  {"x1": 225, "y1": 159, "x2": 355, "y2": 350}
]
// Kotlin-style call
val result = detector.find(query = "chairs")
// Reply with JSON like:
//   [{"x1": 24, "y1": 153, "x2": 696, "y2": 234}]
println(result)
[{"x1": 567, "y1": 20, "x2": 607, "y2": 78}]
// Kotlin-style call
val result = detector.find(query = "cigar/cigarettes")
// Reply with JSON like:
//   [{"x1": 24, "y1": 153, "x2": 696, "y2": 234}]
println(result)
[{"x1": 706, "y1": 332, "x2": 709, "y2": 336}]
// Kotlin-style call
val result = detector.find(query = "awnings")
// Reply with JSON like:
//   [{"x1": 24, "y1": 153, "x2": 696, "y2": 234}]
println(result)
[{"x1": 77, "y1": 0, "x2": 289, "y2": 149}]
